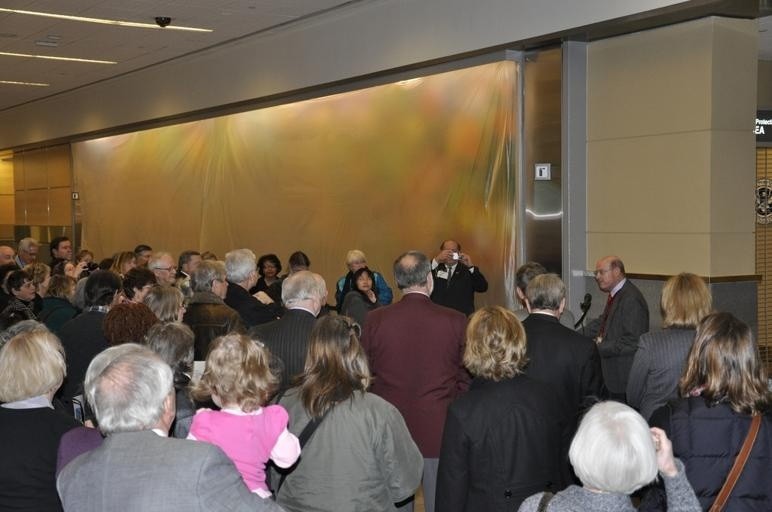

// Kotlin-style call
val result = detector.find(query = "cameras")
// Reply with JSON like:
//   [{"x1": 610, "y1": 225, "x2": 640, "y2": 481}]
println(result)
[
  {"x1": 86, "y1": 263, "x2": 103, "y2": 273},
  {"x1": 453, "y1": 253, "x2": 465, "y2": 261}
]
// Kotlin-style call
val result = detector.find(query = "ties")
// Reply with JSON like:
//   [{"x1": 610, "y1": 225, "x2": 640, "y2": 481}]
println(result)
[
  {"x1": 599, "y1": 293, "x2": 613, "y2": 338},
  {"x1": 447, "y1": 267, "x2": 453, "y2": 289}
]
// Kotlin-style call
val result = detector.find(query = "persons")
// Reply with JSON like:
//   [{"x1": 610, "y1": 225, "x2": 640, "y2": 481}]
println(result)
[
  {"x1": 54, "y1": 270, "x2": 125, "y2": 397},
  {"x1": 340, "y1": 269, "x2": 379, "y2": 316},
  {"x1": 435, "y1": 305, "x2": 569, "y2": 511},
  {"x1": 134, "y1": 245, "x2": 152, "y2": 268},
  {"x1": 247, "y1": 253, "x2": 282, "y2": 295},
  {"x1": 110, "y1": 251, "x2": 136, "y2": 280},
  {"x1": 142, "y1": 322, "x2": 195, "y2": 438},
  {"x1": 626, "y1": 271, "x2": 713, "y2": 425},
  {"x1": 45, "y1": 236, "x2": 74, "y2": 271},
  {"x1": 200, "y1": 252, "x2": 217, "y2": 264},
  {"x1": 1, "y1": 319, "x2": 82, "y2": 511},
  {"x1": 184, "y1": 261, "x2": 248, "y2": 361},
  {"x1": 39, "y1": 273, "x2": 83, "y2": 331},
  {"x1": 60, "y1": 342, "x2": 288, "y2": 511},
  {"x1": 429, "y1": 239, "x2": 488, "y2": 314},
  {"x1": 574, "y1": 255, "x2": 650, "y2": 398},
  {"x1": 647, "y1": 311, "x2": 771, "y2": 511},
  {"x1": 54, "y1": 261, "x2": 89, "y2": 283},
  {"x1": 15, "y1": 238, "x2": 39, "y2": 269},
  {"x1": 264, "y1": 270, "x2": 323, "y2": 387},
  {"x1": 75, "y1": 250, "x2": 100, "y2": 274},
  {"x1": 335, "y1": 249, "x2": 393, "y2": 309},
  {"x1": 265, "y1": 251, "x2": 311, "y2": 303},
  {"x1": 144, "y1": 286, "x2": 187, "y2": 324},
  {"x1": 21, "y1": 263, "x2": 52, "y2": 315},
  {"x1": 0, "y1": 271, "x2": 37, "y2": 329},
  {"x1": 103, "y1": 303, "x2": 160, "y2": 341},
  {"x1": 175, "y1": 250, "x2": 202, "y2": 284},
  {"x1": 146, "y1": 251, "x2": 178, "y2": 287},
  {"x1": 509, "y1": 263, "x2": 572, "y2": 325},
  {"x1": 123, "y1": 267, "x2": 156, "y2": 302},
  {"x1": 516, "y1": 402, "x2": 705, "y2": 511},
  {"x1": 185, "y1": 332, "x2": 301, "y2": 498},
  {"x1": 224, "y1": 248, "x2": 284, "y2": 327},
  {"x1": 0, "y1": 245, "x2": 16, "y2": 266},
  {"x1": 265, "y1": 315, "x2": 424, "y2": 511},
  {"x1": 361, "y1": 251, "x2": 467, "y2": 512}
]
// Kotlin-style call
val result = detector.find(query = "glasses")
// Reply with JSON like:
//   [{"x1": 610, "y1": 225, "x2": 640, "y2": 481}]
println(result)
[
  {"x1": 154, "y1": 265, "x2": 177, "y2": 272},
  {"x1": 594, "y1": 268, "x2": 613, "y2": 274},
  {"x1": 177, "y1": 302, "x2": 186, "y2": 310}
]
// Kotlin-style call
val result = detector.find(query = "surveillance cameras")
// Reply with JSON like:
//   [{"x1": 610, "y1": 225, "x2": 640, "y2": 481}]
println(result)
[{"x1": 154, "y1": 16, "x2": 172, "y2": 28}]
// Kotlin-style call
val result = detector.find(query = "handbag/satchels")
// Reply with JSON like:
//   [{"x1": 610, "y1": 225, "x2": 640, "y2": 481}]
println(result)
[
  {"x1": 272, "y1": 389, "x2": 349, "y2": 498},
  {"x1": 707, "y1": 411, "x2": 760, "y2": 512}
]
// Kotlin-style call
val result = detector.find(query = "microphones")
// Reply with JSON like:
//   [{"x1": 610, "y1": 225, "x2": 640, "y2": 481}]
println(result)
[{"x1": 575, "y1": 293, "x2": 592, "y2": 333}]
